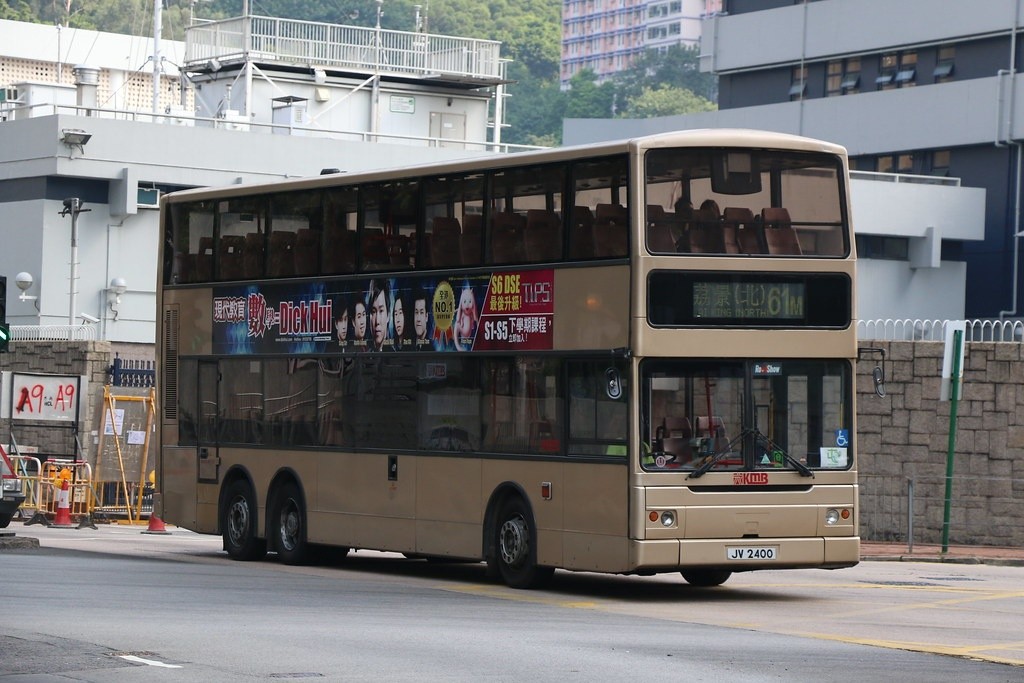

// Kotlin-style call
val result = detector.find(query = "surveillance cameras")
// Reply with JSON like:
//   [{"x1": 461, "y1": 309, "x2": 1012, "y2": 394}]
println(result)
[{"x1": 81, "y1": 313, "x2": 101, "y2": 323}]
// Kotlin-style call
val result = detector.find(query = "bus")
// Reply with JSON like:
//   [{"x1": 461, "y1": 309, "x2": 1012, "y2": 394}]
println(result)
[{"x1": 150, "y1": 126, "x2": 886, "y2": 586}]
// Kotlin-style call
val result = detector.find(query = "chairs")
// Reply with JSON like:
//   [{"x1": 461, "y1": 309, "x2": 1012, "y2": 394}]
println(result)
[
  {"x1": 196, "y1": 203, "x2": 803, "y2": 284},
  {"x1": 662, "y1": 416, "x2": 732, "y2": 463}
]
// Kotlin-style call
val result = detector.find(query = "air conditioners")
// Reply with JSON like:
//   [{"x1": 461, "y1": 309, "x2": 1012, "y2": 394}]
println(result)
[{"x1": 137, "y1": 187, "x2": 161, "y2": 209}]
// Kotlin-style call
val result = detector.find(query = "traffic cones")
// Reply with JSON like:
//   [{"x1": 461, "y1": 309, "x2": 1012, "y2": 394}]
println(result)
[
  {"x1": 48, "y1": 478, "x2": 78, "y2": 529},
  {"x1": 140, "y1": 509, "x2": 174, "y2": 535}
]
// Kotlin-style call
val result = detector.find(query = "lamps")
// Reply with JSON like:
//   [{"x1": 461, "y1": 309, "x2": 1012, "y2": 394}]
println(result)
[
  {"x1": 309, "y1": 66, "x2": 327, "y2": 84},
  {"x1": 208, "y1": 59, "x2": 222, "y2": 72},
  {"x1": 15, "y1": 272, "x2": 40, "y2": 316},
  {"x1": 105, "y1": 276, "x2": 127, "y2": 322},
  {"x1": 59, "y1": 129, "x2": 92, "y2": 145}
]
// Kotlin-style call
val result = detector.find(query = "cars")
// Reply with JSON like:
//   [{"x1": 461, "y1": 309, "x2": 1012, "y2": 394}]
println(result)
[{"x1": 0, "y1": 459, "x2": 24, "y2": 528}]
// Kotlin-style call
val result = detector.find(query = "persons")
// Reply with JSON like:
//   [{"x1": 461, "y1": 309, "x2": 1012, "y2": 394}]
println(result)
[
  {"x1": 367, "y1": 279, "x2": 395, "y2": 352},
  {"x1": 349, "y1": 294, "x2": 367, "y2": 353},
  {"x1": 393, "y1": 291, "x2": 412, "y2": 351},
  {"x1": 325, "y1": 299, "x2": 349, "y2": 353},
  {"x1": 413, "y1": 291, "x2": 436, "y2": 351}
]
